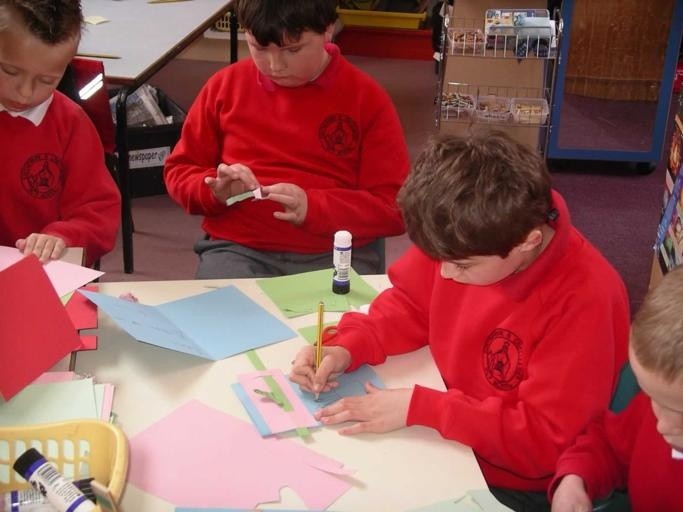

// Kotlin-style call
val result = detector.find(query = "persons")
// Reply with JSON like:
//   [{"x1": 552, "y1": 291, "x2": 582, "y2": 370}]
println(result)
[
  {"x1": 286, "y1": 122, "x2": 632, "y2": 510},
  {"x1": 0, "y1": 0, "x2": 122, "y2": 269},
  {"x1": 543, "y1": 261, "x2": 682, "y2": 510},
  {"x1": 162, "y1": 1, "x2": 414, "y2": 276}
]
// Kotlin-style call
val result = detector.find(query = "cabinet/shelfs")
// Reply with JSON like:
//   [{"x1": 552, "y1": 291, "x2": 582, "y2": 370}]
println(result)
[{"x1": 433, "y1": 4, "x2": 564, "y2": 167}]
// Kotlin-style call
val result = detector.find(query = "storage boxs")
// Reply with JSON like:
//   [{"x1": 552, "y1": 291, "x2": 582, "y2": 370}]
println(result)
[
  {"x1": 102, "y1": 84, "x2": 187, "y2": 200},
  {"x1": 335, "y1": 5, "x2": 429, "y2": 30}
]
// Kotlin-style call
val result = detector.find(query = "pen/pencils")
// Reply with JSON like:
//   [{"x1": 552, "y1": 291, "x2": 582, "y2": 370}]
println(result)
[
  {"x1": 76, "y1": 53, "x2": 122, "y2": 59},
  {"x1": 315, "y1": 300, "x2": 325, "y2": 401}
]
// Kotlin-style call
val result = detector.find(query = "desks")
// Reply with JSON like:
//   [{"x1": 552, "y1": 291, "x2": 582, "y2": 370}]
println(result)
[
  {"x1": 69, "y1": 1, "x2": 239, "y2": 274},
  {"x1": 40, "y1": 271, "x2": 492, "y2": 512}
]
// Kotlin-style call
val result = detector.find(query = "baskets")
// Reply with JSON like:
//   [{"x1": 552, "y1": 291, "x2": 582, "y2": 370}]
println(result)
[
  {"x1": 0, "y1": 421, "x2": 128, "y2": 512},
  {"x1": 216, "y1": 12, "x2": 246, "y2": 33}
]
